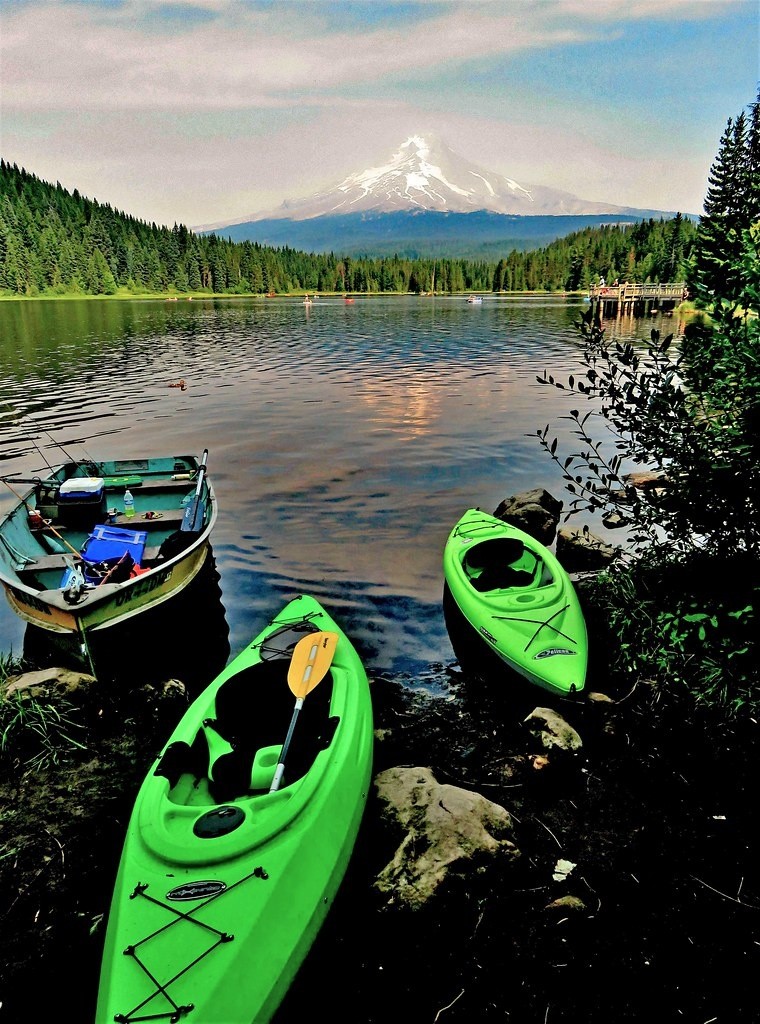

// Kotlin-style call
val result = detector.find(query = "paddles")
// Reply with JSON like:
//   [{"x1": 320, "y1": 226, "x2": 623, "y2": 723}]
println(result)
[
  {"x1": 180, "y1": 448, "x2": 210, "y2": 533},
  {"x1": 267, "y1": 629, "x2": 341, "y2": 792}
]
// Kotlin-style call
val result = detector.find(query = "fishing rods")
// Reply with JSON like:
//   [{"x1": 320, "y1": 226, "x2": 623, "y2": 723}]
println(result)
[
  {"x1": 63, "y1": 429, "x2": 108, "y2": 477},
  {"x1": 1, "y1": 481, "x2": 110, "y2": 577},
  {"x1": 2, "y1": 397, "x2": 62, "y2": 481},
  {"x1": 17, "y1": 408, "x2": 90, "y2": 477}
]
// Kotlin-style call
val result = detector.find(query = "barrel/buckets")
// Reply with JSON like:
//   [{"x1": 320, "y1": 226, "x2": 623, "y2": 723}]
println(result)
[
  {"x1": 29, "y1": 510, "x2": 41, "y2": 529},
  {"x1": 84, "y1": 556, "x2": 135, "y2": 586}
]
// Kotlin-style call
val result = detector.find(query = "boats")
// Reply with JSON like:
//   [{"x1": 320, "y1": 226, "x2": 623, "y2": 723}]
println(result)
[
  {"x1": 465, "y1": 296, "x2": 484, "y2": 305},
  {"x1": 90, "y1": 590, "x2": 377, "y2": 1024},
  {"x1": 0, "y1": 453, "x2": 219, "y2": 663},
  {"x1": 440, "y1": 504, "x2": 589, "y2": 697}
]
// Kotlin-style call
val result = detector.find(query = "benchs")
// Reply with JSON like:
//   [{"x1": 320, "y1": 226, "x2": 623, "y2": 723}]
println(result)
[
  {"x1": 106, "y1": 476, "x2": 197, "y2": 491},
  {"x1": 15, "y1": 544, "x2": 166, "y2": 570},
  {"x1": 109, "y1": 508, "x2": 186, "y2": 527}
]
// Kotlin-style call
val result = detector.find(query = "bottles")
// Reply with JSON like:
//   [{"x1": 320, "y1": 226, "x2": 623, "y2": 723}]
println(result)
[
  {"x1": 124, "y1": 490, "x2": 136, "y2": 519},
  {"x1": 189, "y1": 470, "x2": 196, "y2": 480},
  {"x1": 171, "y1": 474, "x2": 189, "y2": 480}
]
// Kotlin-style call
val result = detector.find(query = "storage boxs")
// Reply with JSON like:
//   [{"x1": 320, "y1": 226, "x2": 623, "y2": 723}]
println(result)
[{"x1": 59, "y1": 477, "x2": 105, "y2": 500}]
